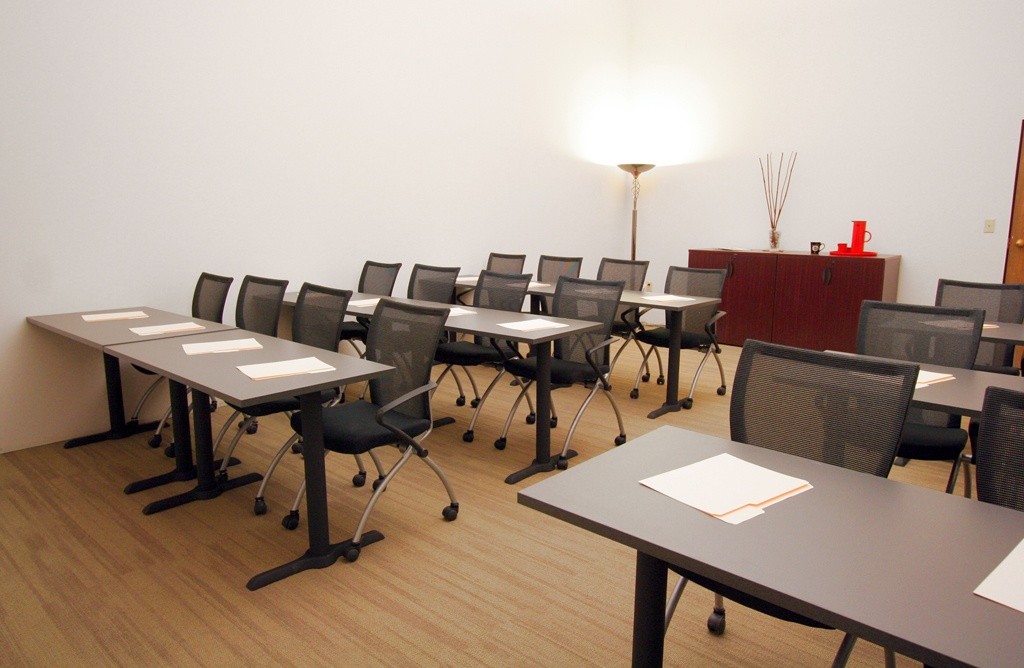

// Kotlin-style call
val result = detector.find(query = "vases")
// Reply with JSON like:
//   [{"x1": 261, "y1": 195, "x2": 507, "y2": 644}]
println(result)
[{"x1": 767, "y1": 226, "x2": 781, "y2": 249}]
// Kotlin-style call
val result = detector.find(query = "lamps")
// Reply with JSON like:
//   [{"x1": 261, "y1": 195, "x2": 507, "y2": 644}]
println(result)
[{"x1": 614, "y1": 161, "x2": 655, "y2": 331}]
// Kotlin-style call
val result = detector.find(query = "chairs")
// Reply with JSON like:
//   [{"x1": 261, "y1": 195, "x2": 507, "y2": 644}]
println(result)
[{"x1": 26, "y1": 247, "x2": 1022, "y2": 666}]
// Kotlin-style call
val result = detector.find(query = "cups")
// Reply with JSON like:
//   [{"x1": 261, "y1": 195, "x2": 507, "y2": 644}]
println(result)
[
  {"x1": 838, "y1": 243, "x2": 848, "y2": 253},
  {"x1": 851, "y1": 220, "x2": 871, "y2": 252},
  {"x1": 810, "y1": 241, "x2": 825, "y2": 254}
]
